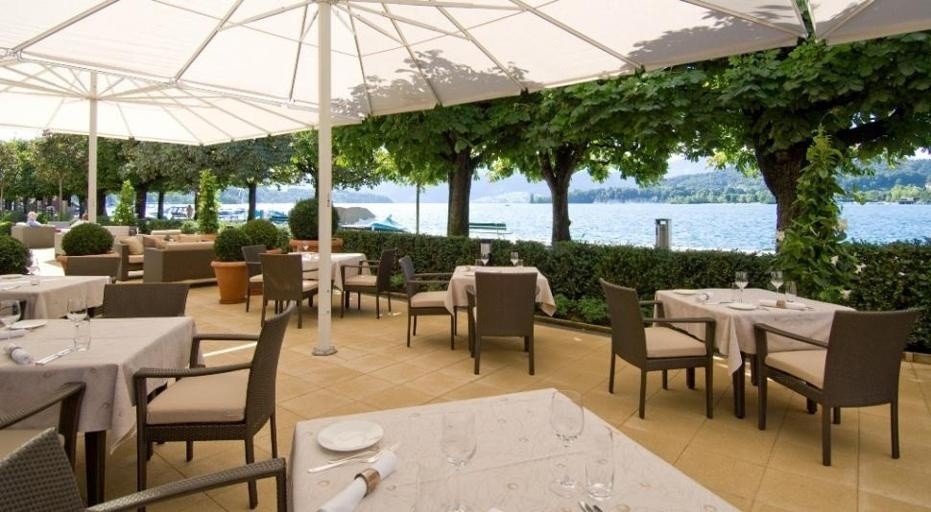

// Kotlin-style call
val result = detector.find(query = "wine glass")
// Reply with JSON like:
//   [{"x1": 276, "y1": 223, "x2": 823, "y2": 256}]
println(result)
[
  {"x1": 736, "y1": 270, "x2": 750, "y2": 305},
  {"x1": 295, "y1": 242, "x2": 319, "y2": 258},
  {"x1": 1, "y1": 298, "x2": 23, "y2": 354},
  {"x1": 771, "y1": 271, "x2": 784, "y2": 306},
  {"x1": 24, "y1": 250, "x2": 43, "y2": 289},
  {"x1": 411, "y1": 392, "x2": 624, "y2": 512},
  {"x1": 474, "y1": 253, "x2": 490, "y2": 270},
  {"x1": 67, "y1": 294, "x2": 87, "y2": 355},
  {"x1": 163, "y1": 232, "x2": 206, "y2": 245},
  {"x1": 511, "y1": 251, "x2": 525, "y2": 272}
]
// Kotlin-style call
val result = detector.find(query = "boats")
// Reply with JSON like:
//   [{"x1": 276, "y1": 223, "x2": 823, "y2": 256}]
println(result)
[
  {"x1": 340, "y1": 212, "x2": 410, "y2": 233},
  {"x1": 72, "y1": 197, "x2": 287, "y2": 226},
  {"x1": 469, "y1": 221, "x2": 514, "y2": 234}
]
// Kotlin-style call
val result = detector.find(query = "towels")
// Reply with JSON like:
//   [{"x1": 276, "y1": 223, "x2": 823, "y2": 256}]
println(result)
[
  {"x1": 695, "y1": 291, "x2": 713, "y2": 303},
  {"x1": 757, "y1": 298, "x2": 814, "y2": 310}
]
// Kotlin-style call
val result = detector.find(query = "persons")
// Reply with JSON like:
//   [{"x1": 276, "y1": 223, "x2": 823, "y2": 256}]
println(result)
[{"x1": 25, "y1": 210, "x2": 42, "y2": 229}]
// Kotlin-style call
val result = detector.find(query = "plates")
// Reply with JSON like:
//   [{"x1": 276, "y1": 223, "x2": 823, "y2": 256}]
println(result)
[
  {"x1": 463, "y1": 271, "x2": 475, "y2": 278},
  {"x1": 674, "y1": 289, "x2": 703, "y2": 297},
  {"x1": 730, "y1": 302, "x2": 759, "y2": 311},
  {"x1": 10, "y1": 318, "x2": 46, "y2": 331},
  {"x1": 318, "y1": 416, "x2": 385, "y2": 453}
]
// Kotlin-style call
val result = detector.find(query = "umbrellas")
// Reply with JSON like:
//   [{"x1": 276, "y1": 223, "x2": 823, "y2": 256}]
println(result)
[
  {"x1": 0, "y1": 0, "x2": 808, "y2": 354},
  {"x1": 806, "y1": 0, "x2": 930, "y2": 47},
  {"x1": 0, "y1": 54, "x2": 362, "y2": 226}
]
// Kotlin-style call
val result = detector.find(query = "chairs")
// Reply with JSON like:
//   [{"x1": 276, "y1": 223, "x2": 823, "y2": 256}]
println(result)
[
  {"x1": 753, "y1": 305, "x2": 928, "y2": 466},
  {"x1": 599, "y1": 277, "x2": 716, "y2": 418}
]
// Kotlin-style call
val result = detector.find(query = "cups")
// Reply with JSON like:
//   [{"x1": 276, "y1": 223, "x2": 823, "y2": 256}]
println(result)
[
  {"x1": 75, "y1": 312, "x2": 91, "y2": 352},
  {"x1": 786, "y1": 280, "x2": 798, "y2": 302}
]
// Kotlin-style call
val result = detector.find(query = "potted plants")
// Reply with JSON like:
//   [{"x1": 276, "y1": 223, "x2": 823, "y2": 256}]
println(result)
[{"x1": 287, "y1": 198, "x2": 345, "y2": 254}]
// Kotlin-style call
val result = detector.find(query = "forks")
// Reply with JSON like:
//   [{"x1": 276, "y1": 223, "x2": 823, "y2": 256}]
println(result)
[{"x1": 304, "y1": 450, "x2": 387, "y2": 480}]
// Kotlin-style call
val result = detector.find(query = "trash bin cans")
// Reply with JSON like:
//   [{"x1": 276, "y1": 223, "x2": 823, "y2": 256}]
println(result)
[{"x1": 656, "y1": 219, "x2": 670, "y2": 250}]
